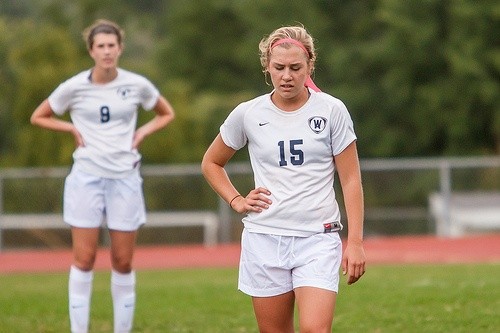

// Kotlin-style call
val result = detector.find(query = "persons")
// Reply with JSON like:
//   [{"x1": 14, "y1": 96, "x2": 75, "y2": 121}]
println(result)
[
  {"x1": 200, "y1": 26, "x2": 366, "y2": 333},
  {"x1": 29, "y1": 19, "x2": 175, "y2": 333}
]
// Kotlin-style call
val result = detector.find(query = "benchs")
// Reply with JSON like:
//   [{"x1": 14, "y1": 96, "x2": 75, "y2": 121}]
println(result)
[
  {"x1": 428, "y1": 191, "x2": 500, "y2": 239},
  {"x1": 0, "y1": 212, "x2": 219, "y2": 248}
]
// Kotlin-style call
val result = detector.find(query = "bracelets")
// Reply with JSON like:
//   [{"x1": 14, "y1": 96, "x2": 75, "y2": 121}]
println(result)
[{"x1": 228, "y1": 194, "x2": 242, "y2": 208}]
